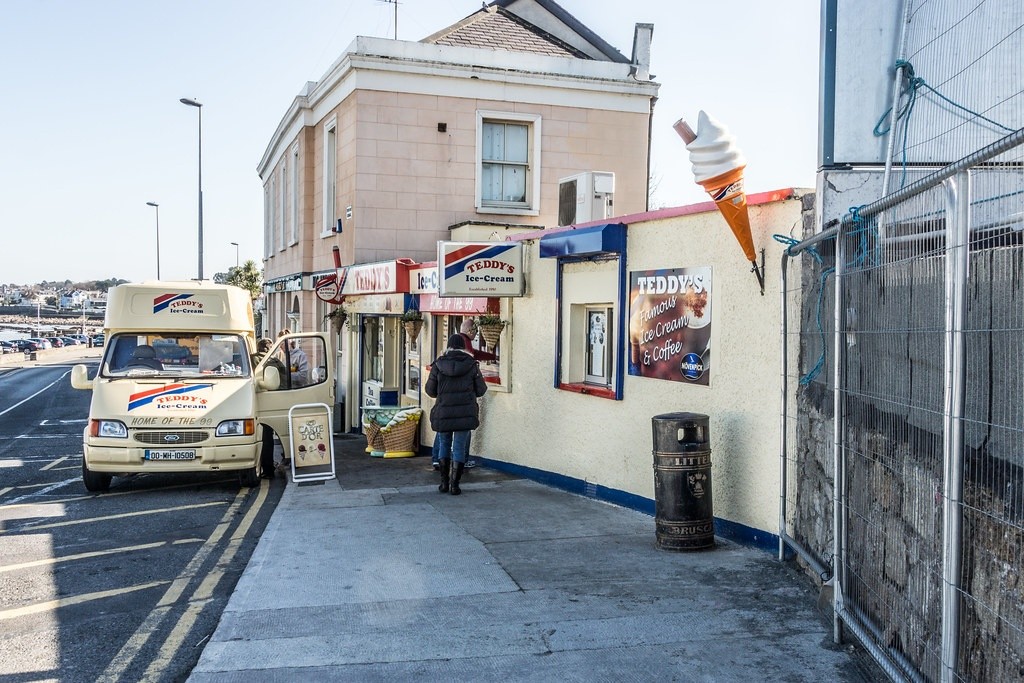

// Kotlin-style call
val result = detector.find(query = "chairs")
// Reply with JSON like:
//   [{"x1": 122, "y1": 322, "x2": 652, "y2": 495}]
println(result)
[{"x1": 122, "y1": 344, "x2": 165, "y2": 371}]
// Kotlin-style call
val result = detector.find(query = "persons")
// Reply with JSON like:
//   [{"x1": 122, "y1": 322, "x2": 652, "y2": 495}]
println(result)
[
  {"x1": 274, "y1": 329, "x2": 308, "y2": 466},
  {"x1": 432, "y1": 319, "x2": 479, "y2": 471},
  {"x1": 250, "y1": 338, "x2": 285, "y2": 478},
  {"x1": 424, "y1": 334, "x2": 487, "y2": 495}
]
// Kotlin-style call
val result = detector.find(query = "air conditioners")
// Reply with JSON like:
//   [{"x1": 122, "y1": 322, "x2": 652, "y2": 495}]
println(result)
[{"x1": 556, "y1": 171, "x2": 616, "y2": 228}]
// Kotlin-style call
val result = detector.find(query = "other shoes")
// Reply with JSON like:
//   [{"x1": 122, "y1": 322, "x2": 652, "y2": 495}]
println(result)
[
  {"x1": 433, "y1": 460, "x2": 439, "y2": 466},
  {"x1": 279, "y1": 453, "x2": 291, "y2": 465},
  {"x1": 260, "y1": 470, "x2": 275, "y2": 479},
  {"x1": 465, "y1": 461, "x2": 476, "y2": 467}
]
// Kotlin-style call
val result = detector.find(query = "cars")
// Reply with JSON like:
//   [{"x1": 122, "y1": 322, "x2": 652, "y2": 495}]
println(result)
[{"x1": 0, "y1": 333, "x2": 105, "y2": 356}]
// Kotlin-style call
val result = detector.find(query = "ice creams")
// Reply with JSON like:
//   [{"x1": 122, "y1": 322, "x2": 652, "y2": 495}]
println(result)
[{"x1": 672, "y1": 109, "x2": 756, "y2": 260}]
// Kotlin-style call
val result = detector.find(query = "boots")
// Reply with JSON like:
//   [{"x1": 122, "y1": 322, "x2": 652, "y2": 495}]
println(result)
[
  {"x1": 450, "y1": 461, "x2": 465, "y2": 494},
  {"x1": 437, "y1": 459, "x2": 450, "y2": 492}
]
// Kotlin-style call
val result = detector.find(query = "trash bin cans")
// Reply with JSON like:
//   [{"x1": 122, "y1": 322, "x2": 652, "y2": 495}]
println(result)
[
  {"x1": 651, "y1": 413, "x2": 714, "y2": 550},
  {"x1": 89, "y1": 338, "x2": 93, "y2": 348}
]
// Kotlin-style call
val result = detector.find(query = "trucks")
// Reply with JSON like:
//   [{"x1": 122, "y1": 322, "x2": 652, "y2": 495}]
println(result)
[{"x1": 70, "y1": 279, "x2": 336, "y2": 494}]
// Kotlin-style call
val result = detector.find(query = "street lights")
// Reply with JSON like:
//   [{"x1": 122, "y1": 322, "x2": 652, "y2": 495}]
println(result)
[
  {"x1": 230, "y1": 242, "x2": 238, "y2": 268},
  {"x1": 144, "y1": 202, "x2": 159, "y2": 280},
  {"x1": 178, "y1": 96, "x2": 206, "y2": 282}
]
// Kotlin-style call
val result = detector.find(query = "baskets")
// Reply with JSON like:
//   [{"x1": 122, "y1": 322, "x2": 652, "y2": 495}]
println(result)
[
  {"x1": 478, "y1": 322, "x2": 503, "y2": 348},
  {"x1": 364, "y1": 418, "x2": 385, "y2": 451},
  {"x1": 330, "y1": 312, "x2": 347, "y2": 335},
  {"x1": 401, "y1": 318, "x2": 424, "y2": 343},
  {"x1": 381, "y1": 418, "x2": 416, "y2": 452}
]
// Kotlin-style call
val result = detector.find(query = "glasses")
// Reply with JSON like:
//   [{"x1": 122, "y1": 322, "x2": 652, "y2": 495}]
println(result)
[{"x1": 469, "y1": 326, "x2": 478, "y2": 332}]
